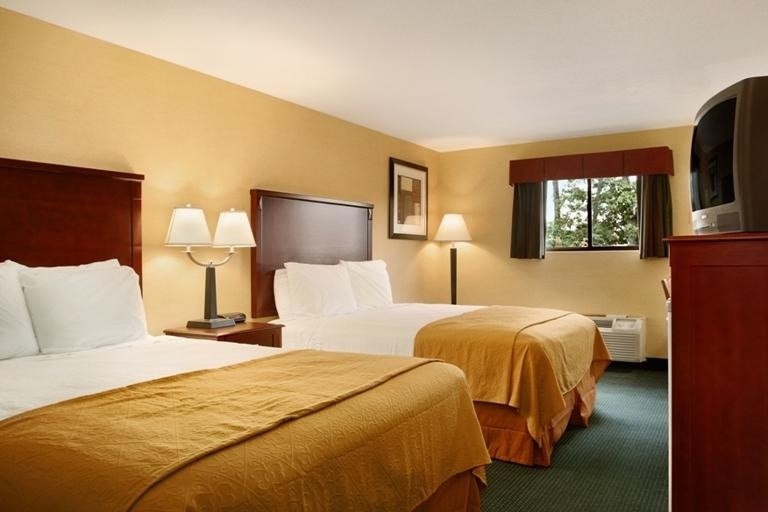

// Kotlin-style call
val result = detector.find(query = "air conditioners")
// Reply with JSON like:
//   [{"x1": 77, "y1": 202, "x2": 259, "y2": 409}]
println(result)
[{"x1": 582, "y1": 314, "x2": 647, "y2": 362}]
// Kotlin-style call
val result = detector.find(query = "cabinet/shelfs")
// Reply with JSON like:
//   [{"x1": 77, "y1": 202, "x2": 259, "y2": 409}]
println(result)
[{"x1": 661, "y1": 235, "x2": 767, "y2": 512}]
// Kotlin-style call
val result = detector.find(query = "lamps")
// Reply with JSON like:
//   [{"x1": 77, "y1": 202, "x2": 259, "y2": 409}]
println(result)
[
  {"x1": 434, "y1": 214, "x2": 473, "y2": 305},
  {"x1": 164, "y1": 204, "x2": 256, "y2": 329}
]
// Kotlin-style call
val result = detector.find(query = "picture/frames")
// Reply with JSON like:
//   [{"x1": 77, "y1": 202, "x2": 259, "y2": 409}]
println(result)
[{"x1": 389, "y1": 157, "x2": 428, "y2": 240}]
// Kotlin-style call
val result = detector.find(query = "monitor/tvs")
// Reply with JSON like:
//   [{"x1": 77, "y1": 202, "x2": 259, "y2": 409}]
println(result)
[{"x1": 688, "y1": 76, "x2": 767, "y2": 235}]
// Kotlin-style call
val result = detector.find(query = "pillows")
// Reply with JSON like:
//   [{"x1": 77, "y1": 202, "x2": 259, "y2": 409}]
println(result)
[
  {"x1": 273, "y1": 259, "x2": 393, "y2": 322},
  {"x1": 1, "y1": 259, "x2": 149, "y2": 360}
]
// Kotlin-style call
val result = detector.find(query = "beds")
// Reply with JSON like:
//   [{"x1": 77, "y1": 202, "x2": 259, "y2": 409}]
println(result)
[
  {"x1": 0, "y1": 157, "x2": 493, "y2": 511},
  {"x1": 250, "y1": 189, "x2": 611, "y2": 467}
]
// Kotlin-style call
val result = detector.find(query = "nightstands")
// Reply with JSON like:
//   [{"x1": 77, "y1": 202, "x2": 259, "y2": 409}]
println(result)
[{"x1": 163, "y1": 322, "x2": 285, "y2": 347}]
